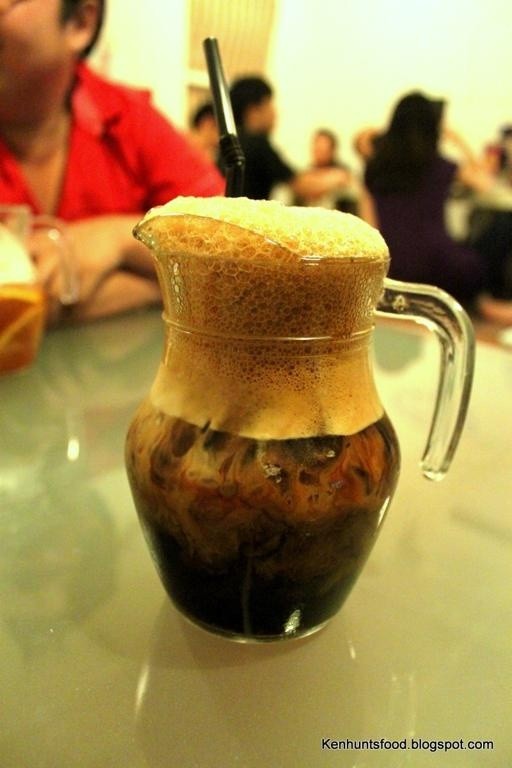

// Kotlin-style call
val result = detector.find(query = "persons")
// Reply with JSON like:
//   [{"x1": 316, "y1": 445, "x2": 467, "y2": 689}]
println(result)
[
  {"x1": 1, "y1": 1, "x2": 235, "y2": 331},
  {"x1": 215, "y1": 69, "x2": 353, "y2": 204},
  {"x1": 355, "y1": 90, "x2": 511, "y2": 333},
  {"x1": 291, "y1": 127, "x2": 359, "y2": 217}
]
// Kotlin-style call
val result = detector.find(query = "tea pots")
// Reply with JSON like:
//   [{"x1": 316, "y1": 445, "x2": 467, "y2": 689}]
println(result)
[{"x1": 119, "y1": 205, "x2": 478, "y2": 650}]
